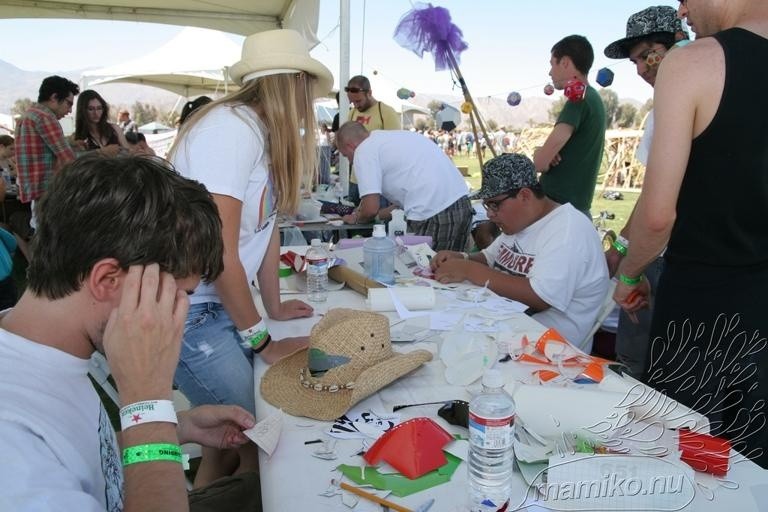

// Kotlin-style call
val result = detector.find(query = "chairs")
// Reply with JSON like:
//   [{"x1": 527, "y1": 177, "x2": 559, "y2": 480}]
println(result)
[{"x1": 578, "y1": 226, "x2": 630, "y2": 351}]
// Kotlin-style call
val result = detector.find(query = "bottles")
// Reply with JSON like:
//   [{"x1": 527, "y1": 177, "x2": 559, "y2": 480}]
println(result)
[
  {"x1": 388, "y1": 209, "x2": 407, "y2": 241},
  {"x1": 2, "y1": 168, "x2": 12, "y2": 189},
  {"x1": 306, "y1": 238, "x2": 328, "y2": 302},
  {"x1": 468, "y1": 369, "x2": 517, "y2": 512},
  {"x1": 362, "y1": 224, "x2": 396, "y2": 286},
  {"x1": 333, "y1": 182, "x2": 344, "y2": 201}
]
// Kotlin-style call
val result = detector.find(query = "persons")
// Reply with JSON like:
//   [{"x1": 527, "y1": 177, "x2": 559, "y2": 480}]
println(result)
[
  {"x1": 470, "y1": 221, "x2": 501, "y2": 253},
  {"x1": 117, "y1": 109, "x2": 155, "y2": 154},
  {"x1": 166, "y1": 29, "x2": 335, "y2": 491},
  {"x1": 317, "y1": 124, "x2": 331, "y2": 184},
  {"x1": 530, "y1": 34, "x2": 606, "y2": 223},
  {"x1": 414, "y1": 126, "x2": 518, "y2": 158},
  {"x1": 612, "y1": 0, "x2": 768, "y2": 470},
  {"x1": 177, "y1": 95, "x2": 212, "y2": 125},
  {"x1": 0, "y1": 147, "x2": 257, "y2": 512},
  {"x1": 76, "y1": 90, "x2": 155, "y2": 158},
  {"x1": 16, "y1": 74, "x2": 120, "y2": 229},
  {"x1": 0, "y1": 134, "x2": 41, "y2": 313},
  {"x1": 336, "y1": 121, "x2": 477, "y2": 253},
  {"x1": 300, "y1": 99, "x2": 343, "y2": 124},
  {"x1": 344, "y1": 75, "x2": 402, "y2": 237},
  {"x1": 428, "y1": 152, "x2": 610, "y2": 356},
  {"x1": 602, "y1": 5, "x2": 690, "y2": 381}
]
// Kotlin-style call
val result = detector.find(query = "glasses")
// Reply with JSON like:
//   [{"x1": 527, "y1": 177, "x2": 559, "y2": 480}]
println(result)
[
  {"x1": 65, "y1": 99, "x2": 74, "y2": 107},
  {"x1": 482, "y1": 195, "x2": 513, "y2": 211},
  {"x1": 344, "y1": 87, "x2": 366, "y2": 93}
]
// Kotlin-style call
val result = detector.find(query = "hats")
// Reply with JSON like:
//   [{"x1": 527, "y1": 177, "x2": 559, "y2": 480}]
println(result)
[
  {"x1": 259, "y1": 307, "x2": 432, "y2": 421},
  {"x1": 467, "y1": 153, "x2": 539, "y2": 200},
  {"x1": 604, "y1": 6, "x2": 689, "y2": 59},
  {"x1": 229, "y1": 29, "x2": 334, "y2": 100}
]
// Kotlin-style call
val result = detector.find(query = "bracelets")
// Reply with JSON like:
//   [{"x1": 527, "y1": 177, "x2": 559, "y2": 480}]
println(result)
[
  {"x1": 461, "y1": 252, "x2": 470, "y2": 259},
  {"x1": 236, "y1": 315, "x2": 271, "y2": 354},
  {"x1": 617, "y1": 234, "x2": 629, "y2": 248},
  {"x1": 122, "y1": 441, "x2": 184, "y2": 466},
  {"x1": 611, "y1": 241, "x2": 626, "y2": 257},
  {"x1": 119, "y1": 400, "x2": 179, "y2": 430},
  {"x1": 619, "y1": 272, "x2": 644, "y2": 286}
]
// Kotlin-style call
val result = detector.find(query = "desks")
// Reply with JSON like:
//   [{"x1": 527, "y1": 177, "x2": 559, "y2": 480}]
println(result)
[
  {"x1": 280, "y1": 214, "x2": 373, "y2": 243},
  {"x1": 254, "y1": 242, "x2": 767, "y2": 511}
]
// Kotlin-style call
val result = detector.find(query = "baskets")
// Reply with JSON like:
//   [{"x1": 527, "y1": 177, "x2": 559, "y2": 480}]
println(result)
[{"x1": 317, "y1": 194, "x2": 355, "y2": 215}]
